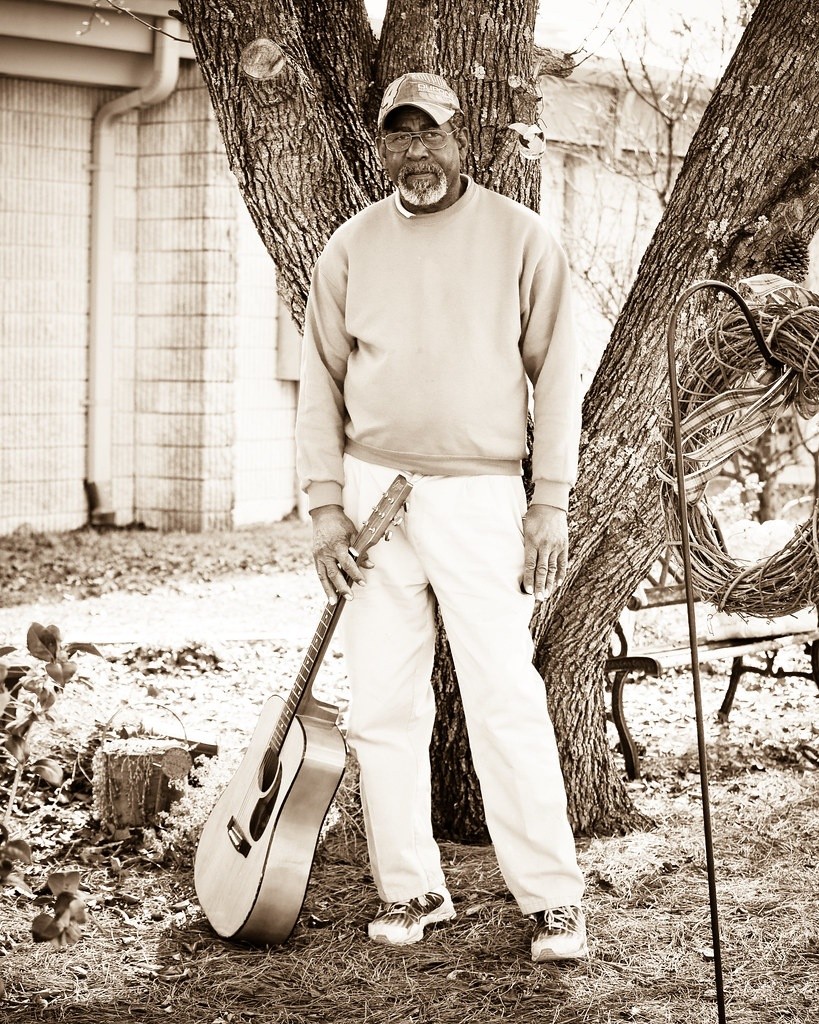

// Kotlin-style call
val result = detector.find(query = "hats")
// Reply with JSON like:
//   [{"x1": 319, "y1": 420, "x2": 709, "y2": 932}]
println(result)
[{"x1": 377, "y1": 73, "x2": 467, "y2": 126}]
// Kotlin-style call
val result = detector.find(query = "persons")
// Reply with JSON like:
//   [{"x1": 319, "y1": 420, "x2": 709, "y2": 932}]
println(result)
[{"x1": 296, "y1": 71, "x2": 589, "y2": 962}]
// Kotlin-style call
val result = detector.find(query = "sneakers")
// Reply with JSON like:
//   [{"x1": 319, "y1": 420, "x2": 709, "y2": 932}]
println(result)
[
  {"x1": 531, "y1": 905, "x2": 586, "y2": 962},
  {"x1": 368, "y1": 884, "x2": 457, "y2": 947}
]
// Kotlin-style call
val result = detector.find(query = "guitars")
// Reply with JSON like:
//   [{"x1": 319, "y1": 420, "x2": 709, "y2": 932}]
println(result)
[{"x1": 194, "y1": 474, "x2": 413, "y2": 950}]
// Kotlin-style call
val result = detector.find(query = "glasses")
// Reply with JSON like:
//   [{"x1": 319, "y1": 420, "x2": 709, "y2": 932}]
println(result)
[{"x1": 381, "y1": 129, "x2": 454, "y2": 153}]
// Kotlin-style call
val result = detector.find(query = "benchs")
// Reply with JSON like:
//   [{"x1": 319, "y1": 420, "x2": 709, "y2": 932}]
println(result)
[{"x1": 602, "y1": 544, "x2": 819, "y2": 780}]
